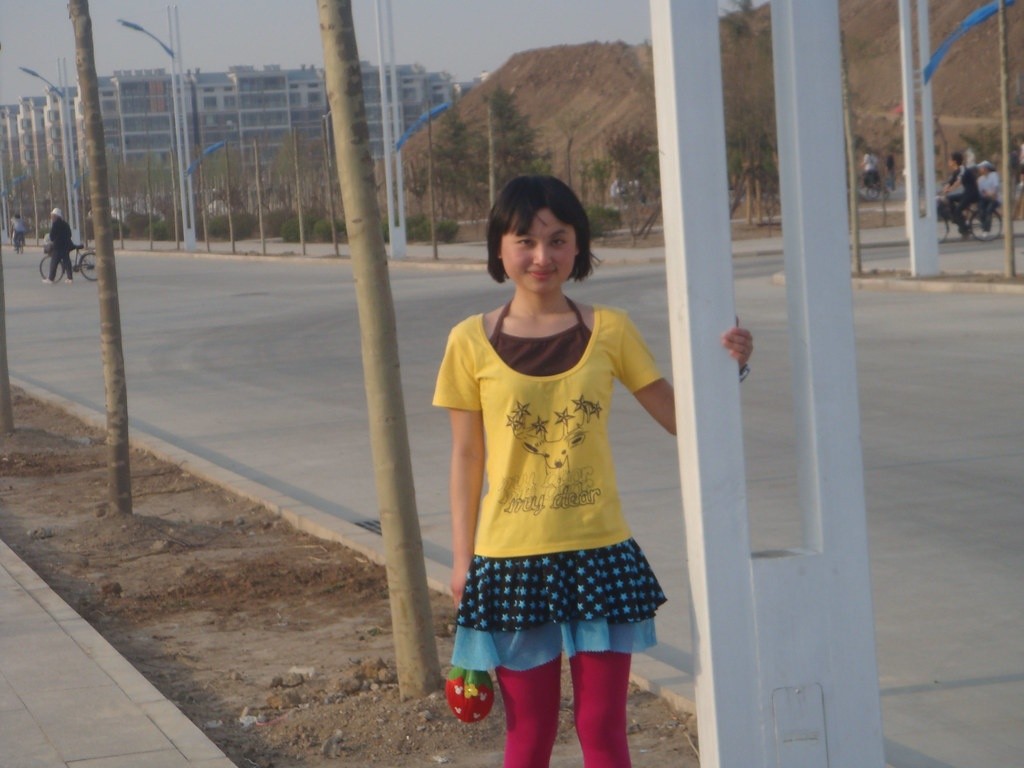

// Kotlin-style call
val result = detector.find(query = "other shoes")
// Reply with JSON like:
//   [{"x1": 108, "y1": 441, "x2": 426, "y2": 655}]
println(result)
[
  {"x1": 960, "y1": 232, "x2": 970, "y2": 239},
  {"x1": 65, "y1": 279, "x2": 73, "y2": 283},
  {"x1": 982, "y1": 231, "x2": 990, "y2": 239},
  {"x1": 41, "y1": 278, "x2": 53, "y2": 284}
]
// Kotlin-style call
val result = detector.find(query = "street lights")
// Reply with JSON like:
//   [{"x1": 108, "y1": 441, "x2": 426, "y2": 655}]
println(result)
[
  {"x1": 18, "y1": 65, "x2": 81, "y2": 246},
  {"x1": 117, "y1": 16, "x2": 196, "y2": 251}
]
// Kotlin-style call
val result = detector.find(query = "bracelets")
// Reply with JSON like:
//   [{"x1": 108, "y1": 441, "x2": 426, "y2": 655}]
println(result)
[{"x1": 739, "y1": 365, "x2": 749, "y2": 381}]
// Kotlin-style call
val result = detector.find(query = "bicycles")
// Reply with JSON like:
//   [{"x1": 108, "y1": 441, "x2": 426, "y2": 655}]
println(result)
[
  {"x1": 935, "y1": 189, "x2": 1003, "y2": 242},
  {"x1": 11, "y1": 235, "x2": 24, "y2": 255},
  {"x1": 864, "y1": 177, "x2": 890, "y2": 201},
  {"x1": 38, "y1": 239, "x2": 99, "y2": 284}
]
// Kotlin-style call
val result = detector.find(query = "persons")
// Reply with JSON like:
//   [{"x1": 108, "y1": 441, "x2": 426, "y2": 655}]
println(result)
[
  {"x1": 10, "y1": 214, "x2": 25, "y2": 251},
  {"x1": 432, "y1": 175, "x2": 754, "y2": 768},
  {"x1": 42, "y1": 208, "x2": 73, "y2": 283},
  {"x1": 1014, "y1": 137, "x2": 1024, "y2": 186},
  {"x1": 937, "y1": 152, "x2": 980, "y2": 233},
  {"x1": 975, "y1": 160, "x2": 1000, "y2": 237},
  {"x1": 885, "y1": 155, "x2": 895, "y2": 190},
  {"x1": 860, "y1": 147, "x2": 880, "y2": 190}
]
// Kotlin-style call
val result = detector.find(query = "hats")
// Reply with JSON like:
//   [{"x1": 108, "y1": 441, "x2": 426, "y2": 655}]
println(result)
[
  {"x1": 50, "y1": 207, "x2": 62, "y2": 216},
  {"x1": 976, "y1": 160, "x2": 994, "y2": 169}
]
